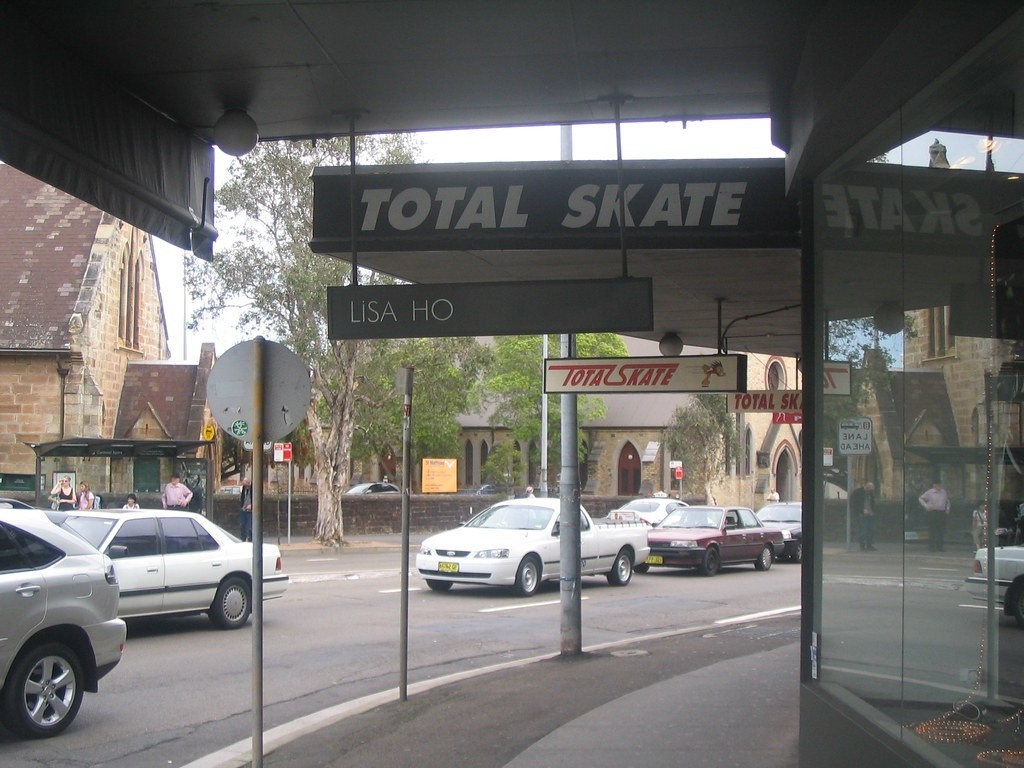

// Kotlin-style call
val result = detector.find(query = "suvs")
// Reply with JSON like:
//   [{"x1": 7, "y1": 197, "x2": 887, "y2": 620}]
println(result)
[{"x1": 1, "y1": 499, "x2": 127, "y2": 738}]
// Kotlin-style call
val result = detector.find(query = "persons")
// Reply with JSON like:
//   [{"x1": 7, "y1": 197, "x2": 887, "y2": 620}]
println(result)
[
  {"x1": 50, "y1": 475, "x2": 77, "y2": 511},
  {"x1": 918, "y1": 478, "x2": 951, "y2": 552},
  {"x1": 526, "y1": 487, "x2": 535, "y2": 498},
  {"x1": 122, "y1": 494, "x2": 139, "y2": 510},
  {"x1": 78, "y1": 480, "x2": 95, "y2": 510},
  {"x1": 239, "y1": 477, "x2": 252, "y2": 542},
  {"x1": 161, "y1": 470, "x2": 204, "y2": 514},
  {"x1": 972, "y1": 498, "x2": 989, "y2": 550},
  {"x1": 767, "y1": 488, "x2": 779, "y2": 504},
  {"x1": 850, "y1": 481, "x2": 878, "y2": 553}
]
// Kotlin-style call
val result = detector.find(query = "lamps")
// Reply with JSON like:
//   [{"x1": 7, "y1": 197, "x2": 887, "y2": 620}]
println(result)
[
  {"x1": 659, "y1": 331, "x2": 683, "y2": 356},
  {"x1": 873, "y1": 301, "x2": 904, "y2": 335},
  {"x1": 212, "y1": 103, "x2": 259, "y2": 156}
]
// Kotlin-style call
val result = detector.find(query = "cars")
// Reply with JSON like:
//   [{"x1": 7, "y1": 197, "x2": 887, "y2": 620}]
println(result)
[
  {"x1": 62, "y1": 509, "x2": 289, "y2": 629},
  {"x1": 459, "y1": 485, "x2": 497, "y2": 495},
  {"x1": 347, "y1": 482, "x2": 401, "y2": 495},
  {"x1": 632, "y1": 506, "x2": 786, "y2": 575},
  {"x1": 756, "y1": 502, "x2": 802, "y2": 563},
  {"x1": 966, "y1": 544, "x2": 1024, "y2": 622},
  {"x1": 606, "y1": 497, "x2": 718, "y2": 526}
]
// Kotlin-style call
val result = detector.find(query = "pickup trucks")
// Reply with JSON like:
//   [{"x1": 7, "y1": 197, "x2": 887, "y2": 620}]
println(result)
[{"x1": 416, "y1": 497, "x2": 651, "y2": 596}]
